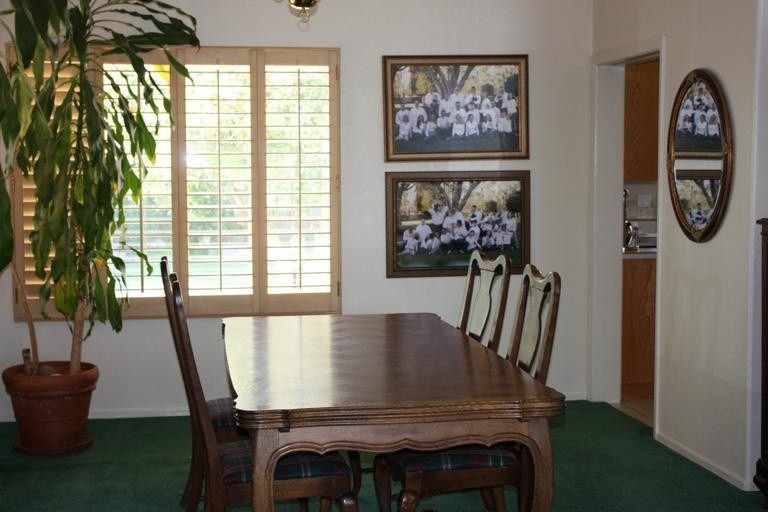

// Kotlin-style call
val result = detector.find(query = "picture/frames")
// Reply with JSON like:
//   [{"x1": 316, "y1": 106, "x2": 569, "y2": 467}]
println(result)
[
  {"x1": 382, "y1": 53, "x2": 531, "y2": 162},
  {"x1": 386, "y1": 172, "x2": 530, "y2": 277},
  {"x1": 675, "y1": 82, "x2": 724, "y2": 229}
]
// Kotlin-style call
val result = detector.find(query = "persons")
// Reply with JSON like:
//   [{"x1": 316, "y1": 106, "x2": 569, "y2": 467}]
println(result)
[
  {"x1": 677, "y1": 87, "x2": 721, "y2": 141},
  {"x1": 686, "y1": 208, "x2": 695, "y2": 227},
  {"x1": 692, "y1": 203, "x2": 706, "y2": 225},
  {"x1": 394, "y1": 83, "x2": 518, "y2": 142},
  {"x1": 397, "y1": 201, "x2": 520, "y2": 257}
]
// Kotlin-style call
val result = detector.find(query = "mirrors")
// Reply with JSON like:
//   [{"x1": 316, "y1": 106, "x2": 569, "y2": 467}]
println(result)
[{"x1": 668, "y1": 69, "x2": 733, "y2": 244}]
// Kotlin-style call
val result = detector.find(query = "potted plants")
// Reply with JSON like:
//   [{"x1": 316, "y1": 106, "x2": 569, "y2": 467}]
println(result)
[{"x1": 1, "y1": 0, "x2": 199, "y2": 461}]
